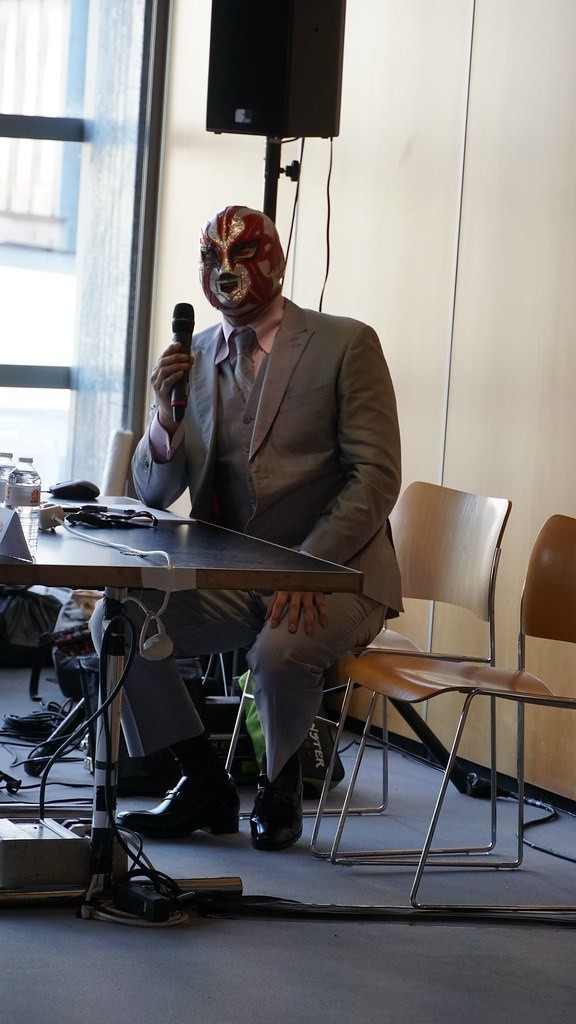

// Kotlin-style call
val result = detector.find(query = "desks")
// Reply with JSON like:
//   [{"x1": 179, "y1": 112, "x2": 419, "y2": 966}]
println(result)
[{"x1": 0, "y1": 497, "x2": 365, "y2": 919}]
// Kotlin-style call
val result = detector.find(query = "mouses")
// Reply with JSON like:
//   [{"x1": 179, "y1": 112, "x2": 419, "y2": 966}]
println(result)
[{"x1": 48, "y1": 480, "x2": 100, "y2": 500}]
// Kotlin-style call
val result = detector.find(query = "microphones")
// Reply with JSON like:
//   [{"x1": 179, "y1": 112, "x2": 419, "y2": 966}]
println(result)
[{"x1": 171, "y1": 302, "x2": 196, "y2": 422}]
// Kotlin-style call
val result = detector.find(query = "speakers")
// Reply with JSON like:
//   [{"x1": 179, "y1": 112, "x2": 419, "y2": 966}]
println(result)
[{"x1": 205, "y1": 0, "x2": 346, "y2": 139}]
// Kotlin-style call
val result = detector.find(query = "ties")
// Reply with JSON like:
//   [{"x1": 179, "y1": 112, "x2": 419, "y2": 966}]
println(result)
[{"x1": 232, "y1": 328, "x2": 257, "y2": 406}]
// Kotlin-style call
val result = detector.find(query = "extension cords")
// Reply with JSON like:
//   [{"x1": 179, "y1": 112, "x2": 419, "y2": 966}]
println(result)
[{"x1": 37, "y1": 501, "x2": 65, "y2": 529}]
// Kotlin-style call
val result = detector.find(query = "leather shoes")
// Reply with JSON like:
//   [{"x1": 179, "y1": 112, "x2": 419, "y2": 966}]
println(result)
[
  {"x1": 118, "y1": 770, "x2": 240, "y2": 840},
  {"x1": 249, "y1": 751, "x2": 305, "y2": 850}
]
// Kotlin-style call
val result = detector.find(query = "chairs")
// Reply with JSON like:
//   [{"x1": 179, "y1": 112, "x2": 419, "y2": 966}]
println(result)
[
  {"x1": 313, "y1": 514, "x2": 576, "y2": 912},
  {"x1": 224, "y1": 482, "x2": 512, "y2": 849}
]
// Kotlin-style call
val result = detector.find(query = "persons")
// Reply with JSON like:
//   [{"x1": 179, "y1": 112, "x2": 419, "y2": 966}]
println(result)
[{"x1": 117, "y1": 205, "x2": 404, "y2": 849}]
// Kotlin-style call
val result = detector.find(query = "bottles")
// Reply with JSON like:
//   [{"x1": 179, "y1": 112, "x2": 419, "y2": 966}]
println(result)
[
  {"x1": 4, "y1": 455, "x2": 42, "y2": 557},
  {"x1": 0, "y1": 450, "x2": 17, "y2": 510}
]
